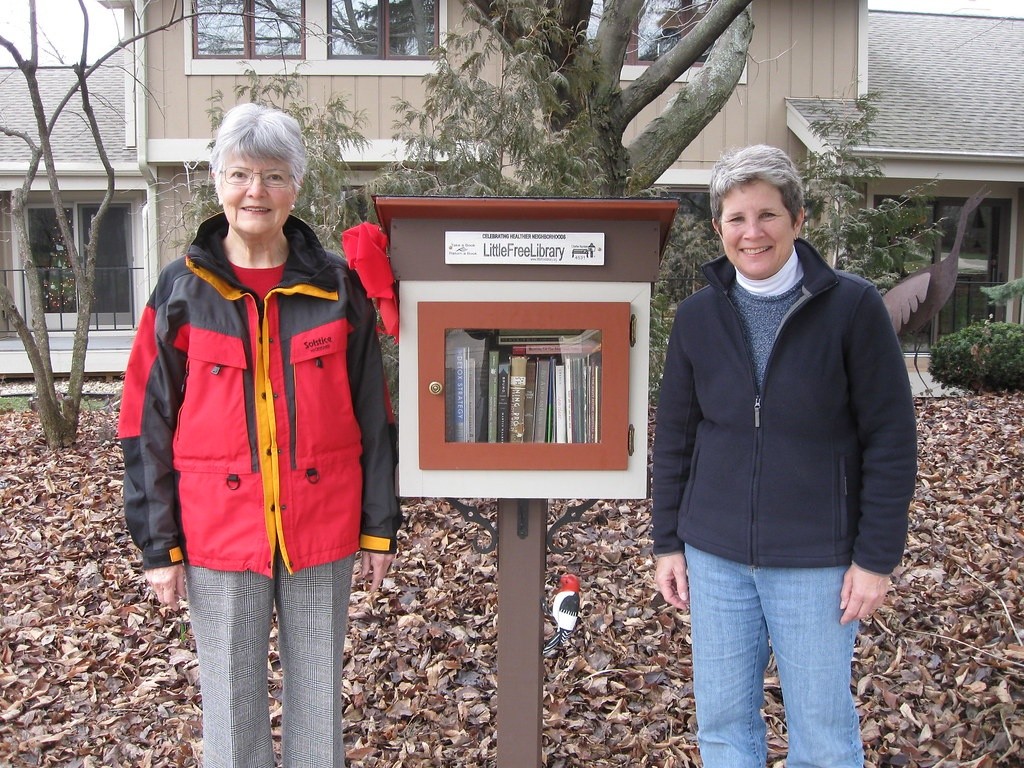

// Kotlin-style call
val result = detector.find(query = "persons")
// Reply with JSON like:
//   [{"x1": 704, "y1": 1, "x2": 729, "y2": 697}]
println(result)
[
  {"x1": 115, "y1": 101, "x2": 401, "y2": 768},
  {"x1": 652, "y1": 144, "x2": 918, "y2": 768}
]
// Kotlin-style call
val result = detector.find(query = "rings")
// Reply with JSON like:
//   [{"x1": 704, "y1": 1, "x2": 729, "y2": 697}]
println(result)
[{"x1": 867, "y1": 614, "x2": 872, "y2": 618}]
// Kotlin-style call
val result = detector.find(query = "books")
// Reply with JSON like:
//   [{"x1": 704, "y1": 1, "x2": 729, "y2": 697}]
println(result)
[{"x1": 444, "y1": 329, "x2": 602, "y2": 444}]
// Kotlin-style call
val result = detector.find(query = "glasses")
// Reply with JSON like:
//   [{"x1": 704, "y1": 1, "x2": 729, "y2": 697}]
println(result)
[{"x1": 220, "y1": 167, "x2": 296, "y2": 187}]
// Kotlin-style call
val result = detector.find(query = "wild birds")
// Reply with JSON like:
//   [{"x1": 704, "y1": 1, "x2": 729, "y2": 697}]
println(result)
[
  {"x1": 881, "y1": 186, "x2": 993, "y2": 398},
  {"x1": 542, "y1": 571, "x2": 581, "y2": 658}
]
